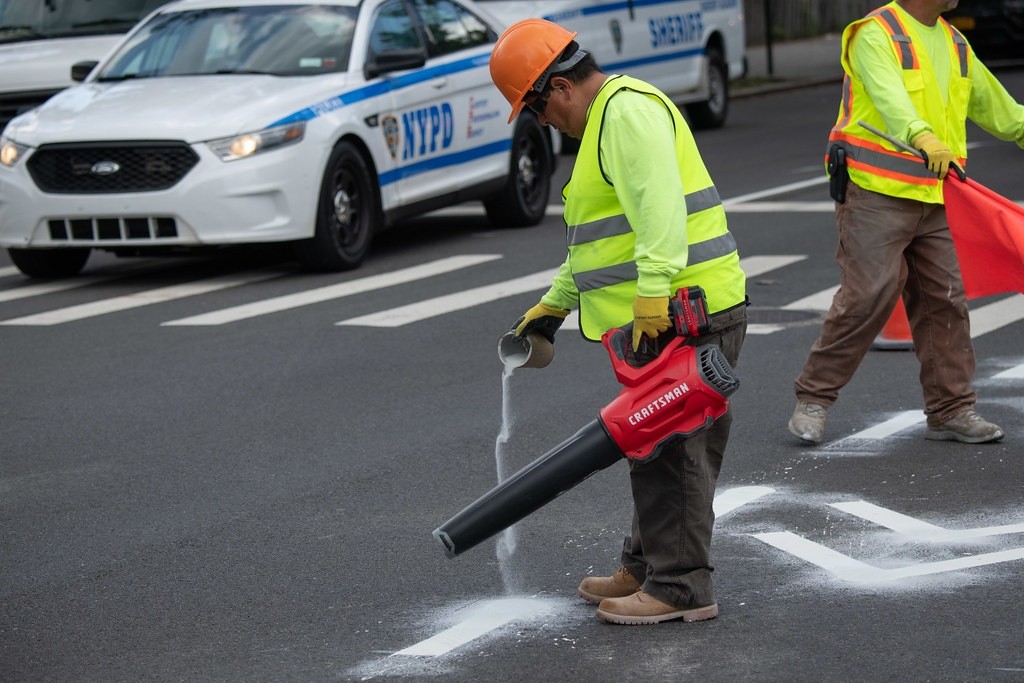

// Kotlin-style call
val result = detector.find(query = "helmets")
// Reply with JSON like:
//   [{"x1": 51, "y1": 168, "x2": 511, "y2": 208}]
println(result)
[{"x1": 489, "y1": 18, "x2": 577, "y2": 125}]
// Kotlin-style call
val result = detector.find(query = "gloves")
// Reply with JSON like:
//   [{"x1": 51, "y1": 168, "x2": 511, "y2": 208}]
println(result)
[
  {"x1": 509, "y1": 302, "x2": 571, "y2": 345},
  {"x1": 913, "y1": 130, "x2": 967, "y2": 183},
  {"x1": 632, "y1": 295, "x2": 673, "y2": 353}
]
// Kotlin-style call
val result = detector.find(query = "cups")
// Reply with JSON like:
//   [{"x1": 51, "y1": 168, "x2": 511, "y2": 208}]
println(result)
[{"x1": 496, "y1": 329, "x2": 555, "y2": 369}]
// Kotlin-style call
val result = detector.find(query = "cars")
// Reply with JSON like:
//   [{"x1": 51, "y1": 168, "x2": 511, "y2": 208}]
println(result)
[
  {"x1": 1, "y1": 1, "x2": 561, "y2": 278},
  {"x1": 430, "y1": 1, "x2": 751, "y2": 171}
]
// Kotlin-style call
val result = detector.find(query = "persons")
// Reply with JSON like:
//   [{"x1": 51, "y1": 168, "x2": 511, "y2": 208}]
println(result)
[
  {"x1": 788, "y1": 1, "x2": 1024, "y2": 445},
  {"x1": 490, "y1": 16, "x2": 746, "y2": 627}
]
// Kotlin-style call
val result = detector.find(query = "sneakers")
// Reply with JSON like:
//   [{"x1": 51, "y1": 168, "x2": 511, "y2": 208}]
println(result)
[
  {"x1": 925, "y1": 405, "x2": 1004, "y2": 443},
  {"x1": 788, "y1": 393, "x2": 828, "y2": 443},
  {"x1": 577, "y1": 564, "x2": 719, "y2": 624}
]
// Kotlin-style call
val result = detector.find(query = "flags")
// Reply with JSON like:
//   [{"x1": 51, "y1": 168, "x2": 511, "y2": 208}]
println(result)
[{"x1": 882, "y1": 168, "x2": 1023, "y2": 339}]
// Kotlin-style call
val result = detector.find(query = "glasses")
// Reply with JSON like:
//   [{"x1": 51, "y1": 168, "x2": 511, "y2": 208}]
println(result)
[{"x1": 528, "y1": 81, "x2": 552, "y2": 117}]
